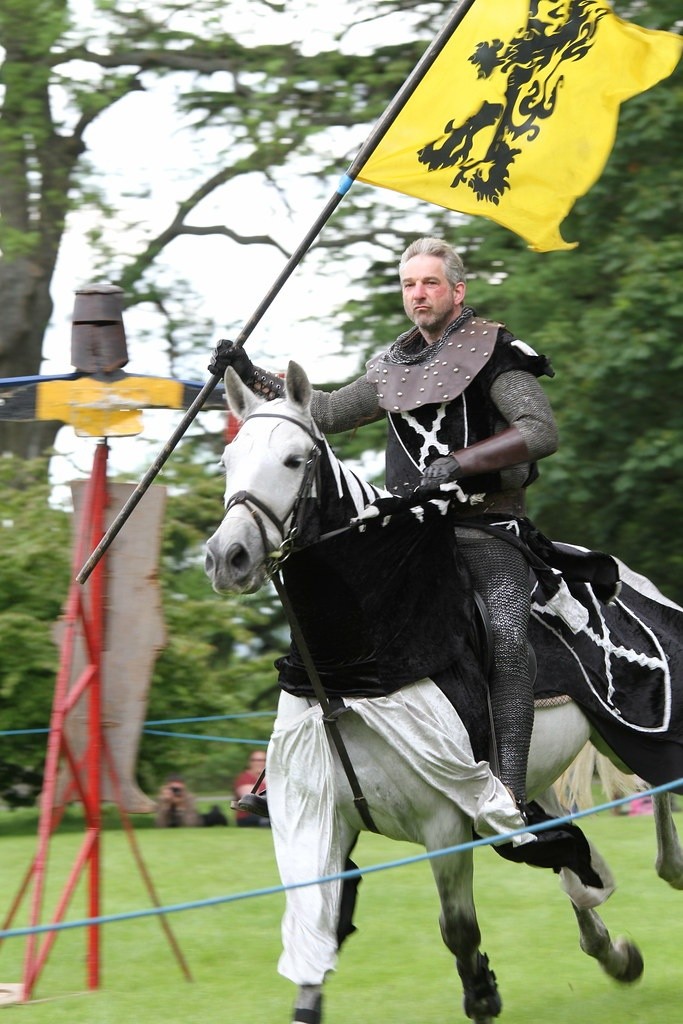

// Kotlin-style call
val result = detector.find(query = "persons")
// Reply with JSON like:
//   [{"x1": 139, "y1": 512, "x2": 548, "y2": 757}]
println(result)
[
  {"x1": 233, "y1": 749, "x2": 271, "y2": 828},
  {"x1": 156, "y1": 776, "x2": 205, "y2": 827},
  {"x1": 208, "y1": 239, "x2": 557, "y2": 844}
]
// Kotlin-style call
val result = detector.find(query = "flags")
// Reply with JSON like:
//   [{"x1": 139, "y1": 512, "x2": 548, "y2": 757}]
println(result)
[{"x1": 354, "y1": 0, "x2": 683, "y2": 253}]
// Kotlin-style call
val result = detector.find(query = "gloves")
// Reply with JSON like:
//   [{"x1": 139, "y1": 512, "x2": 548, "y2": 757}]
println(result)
[
  {"x1": 207, "y1": 338, "x2": 254, "y2": 382},
  {"x1": 418, "y1": 455, "x2": 462, "y2": 491}
]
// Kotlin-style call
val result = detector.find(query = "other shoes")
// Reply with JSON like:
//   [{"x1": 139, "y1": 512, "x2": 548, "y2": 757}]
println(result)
[{"x1": 238, "y1": 792, "x2": 271, "y2": 817}]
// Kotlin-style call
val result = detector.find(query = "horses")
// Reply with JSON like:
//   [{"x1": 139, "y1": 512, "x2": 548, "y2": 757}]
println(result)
[{"x1": 203, "y1": 361, "x2": 683, "y2": 1024}]
